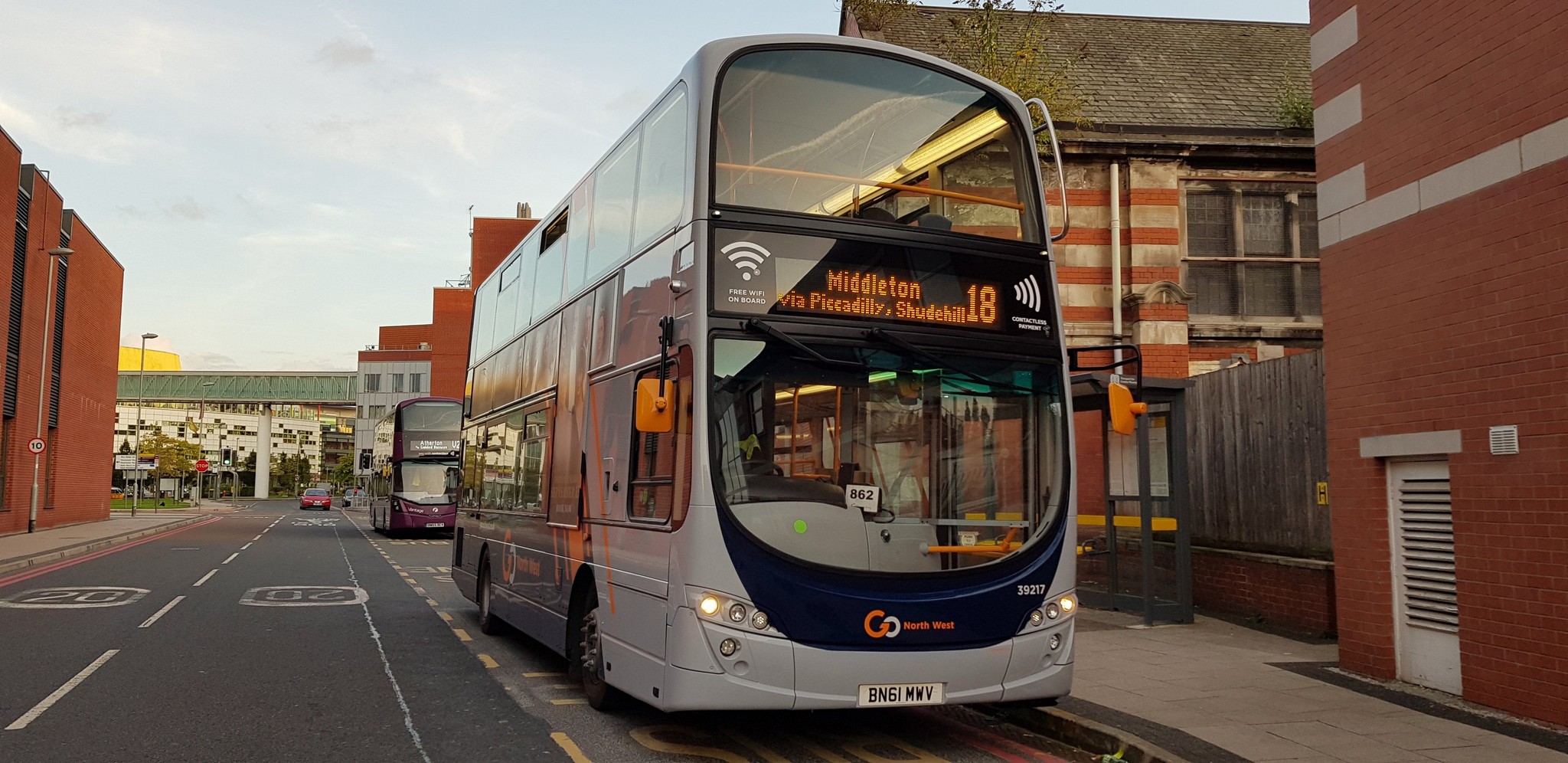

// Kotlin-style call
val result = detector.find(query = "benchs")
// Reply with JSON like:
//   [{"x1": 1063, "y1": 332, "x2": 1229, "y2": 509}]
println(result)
[{"x1": 971, "y1": 530, "x2": 1116, "y2": 561}]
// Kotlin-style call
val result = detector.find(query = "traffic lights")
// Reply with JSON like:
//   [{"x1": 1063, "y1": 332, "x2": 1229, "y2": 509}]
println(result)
[
  {"x1": 222, "y1": 448, "x2": 232, "y2": 466},
  {"x1": 363, "y1": 453, "x2": 372, "y2": 470},
  {"x1": 354, "y1": 489, "x2": 358, "y2": 494}
]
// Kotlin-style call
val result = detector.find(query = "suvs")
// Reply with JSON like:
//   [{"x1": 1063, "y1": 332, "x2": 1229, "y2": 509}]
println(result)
[{"x1": 342, "y1": 486, "x2": 363, "y2": 494}]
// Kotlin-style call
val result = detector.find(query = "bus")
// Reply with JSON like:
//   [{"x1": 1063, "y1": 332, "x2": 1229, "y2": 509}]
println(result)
[
  {"x1": 451, "y1": 35, "x2": 1149, "y2": 712},
  {"x1": 369, "y1": 396, "x2": 464, "y2": 538}
]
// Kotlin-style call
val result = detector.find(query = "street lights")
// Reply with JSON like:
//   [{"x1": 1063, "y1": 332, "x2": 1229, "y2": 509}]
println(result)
[
  {"x1": 219, "y1": 422, "x2": 226, "y2": 449},
  {"x1": 195, "y1": 381, "x2": 215, "y2": 504},
  {"x1": 296, "y1": 431, "x2": 305, "y2": 499},
  {"x1": 132, "y1": 332, "x2": 159, "y2": 517},
  {"x1": 235, "y1": 438, "x2": 240, "y2": 466},
  {"x1": 181, "y1": 406, "x2": 196, "y2": 502}
]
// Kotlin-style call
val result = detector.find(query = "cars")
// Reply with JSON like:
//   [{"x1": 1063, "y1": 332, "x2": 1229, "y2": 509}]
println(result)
[
  {"x1": 111, "y1": 486, "x2": 150, "y2": 499},
  {"x1": 300, "y1": 488, "x2": 332, "y2": 511},
  {"x1": 342, "y1": 489, "x2": 367, "y2": 507}
]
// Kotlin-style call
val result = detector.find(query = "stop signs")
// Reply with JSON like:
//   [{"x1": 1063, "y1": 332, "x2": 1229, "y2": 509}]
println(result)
[{"x1": 195, "y1": 459, "x2": 209, "y2": 472}]
